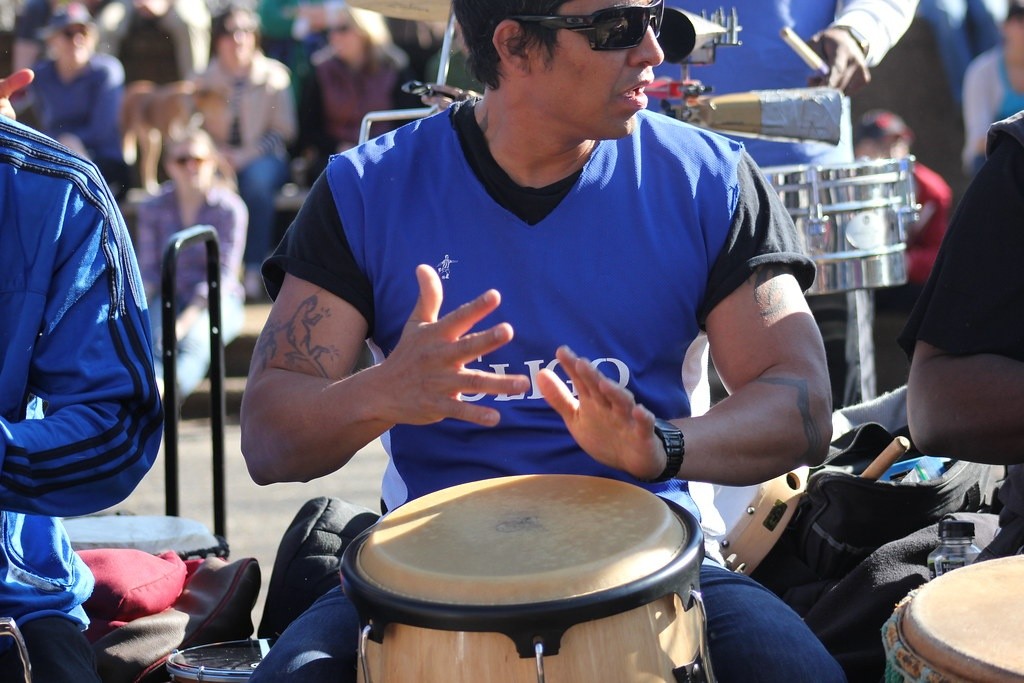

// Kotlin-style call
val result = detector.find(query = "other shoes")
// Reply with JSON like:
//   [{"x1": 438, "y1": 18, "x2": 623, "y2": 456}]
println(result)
[{"x1": 244, "y1": 270, "x2": 264, "y2": 302}]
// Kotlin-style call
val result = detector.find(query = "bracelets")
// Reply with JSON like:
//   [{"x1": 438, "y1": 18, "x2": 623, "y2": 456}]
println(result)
[{"x1": 654, "y1": 418, "x2": 685, "y2": 482}]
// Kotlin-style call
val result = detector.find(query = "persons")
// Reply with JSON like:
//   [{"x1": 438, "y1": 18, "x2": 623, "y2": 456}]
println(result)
[
  {"x1": 1, "y1": 1, "x2": 409, "y2": 297},
  {"x1": 898, "y1": 110, "x2": 1024, "y2": 465},
  {"x1": 0, "y1": 116, "x2": 163, "y2": 682},
  {"x1": 240, "y1": 0, "x2": 848, "y2": 682},
  {"x1": 852, "y1": 110, "x2": 954, "y2": 288},
  {"x1": 645, "y1": 0, "x2": 918, "y2": 182},
  {"x1": 961, "y1": 5, "x2": 1024, "y2": 173},
  {"x1": 135, "y1": 127, "x2": 249, "y2": 402}
]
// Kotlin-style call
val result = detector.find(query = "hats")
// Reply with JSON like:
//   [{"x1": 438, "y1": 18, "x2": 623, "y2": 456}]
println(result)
[
  {"x1": 50, "y1": 3, "x2": 91, "y2": 30},
  {"x1": 856, "y1": 109, "x2": 915, "y2": 141}
]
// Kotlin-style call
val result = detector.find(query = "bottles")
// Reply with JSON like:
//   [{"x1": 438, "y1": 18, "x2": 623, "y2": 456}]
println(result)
[{"x1": 927, "y1": 521, "x2": 981, "y2": 582}]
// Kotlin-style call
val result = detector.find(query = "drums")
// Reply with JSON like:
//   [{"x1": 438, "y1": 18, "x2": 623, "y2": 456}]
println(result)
[
  {"x1": 60, "y1": 513, "x2": 229, "y2": 566},
  {"x1": 711, "y1": 464, "x2": 812, "y2": 578},
  {"x1": 338, "y1": 472, "x2": 721, "y2": 683},
  {"x1": 757, "y1": 152, "x2": 921, "y2": 291},
  {"x1": 878, "y1": 552, "x2": 1024, "y2": 683},
  {"x1": 165, "y1": 637, "x2": 280, "y2": 683}
]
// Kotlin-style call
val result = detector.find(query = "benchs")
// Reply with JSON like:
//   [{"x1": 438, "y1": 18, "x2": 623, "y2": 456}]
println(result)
[{"x1": 188, "y1": 303, "x2": 277, "y2": 393}]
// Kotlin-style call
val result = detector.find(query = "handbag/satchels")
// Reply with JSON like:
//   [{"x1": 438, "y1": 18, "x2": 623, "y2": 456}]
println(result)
[{"x1": 764, "y1": 422, "x2": 992, "y2": 615}]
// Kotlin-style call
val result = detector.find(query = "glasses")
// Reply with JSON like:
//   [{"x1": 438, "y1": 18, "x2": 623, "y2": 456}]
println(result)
[
  {"x1": 224, "y1": 29, "x2": 252, "y2": 38},
  {"x1": 175, "y1": 156, "x2": 205, "y2": 166},
  {"x1": 331, "y1": 25, "x2": 352, "y2": 33},
  {"x1": 506, "y1": 0, "x2": 664, "y2": 50}
]
